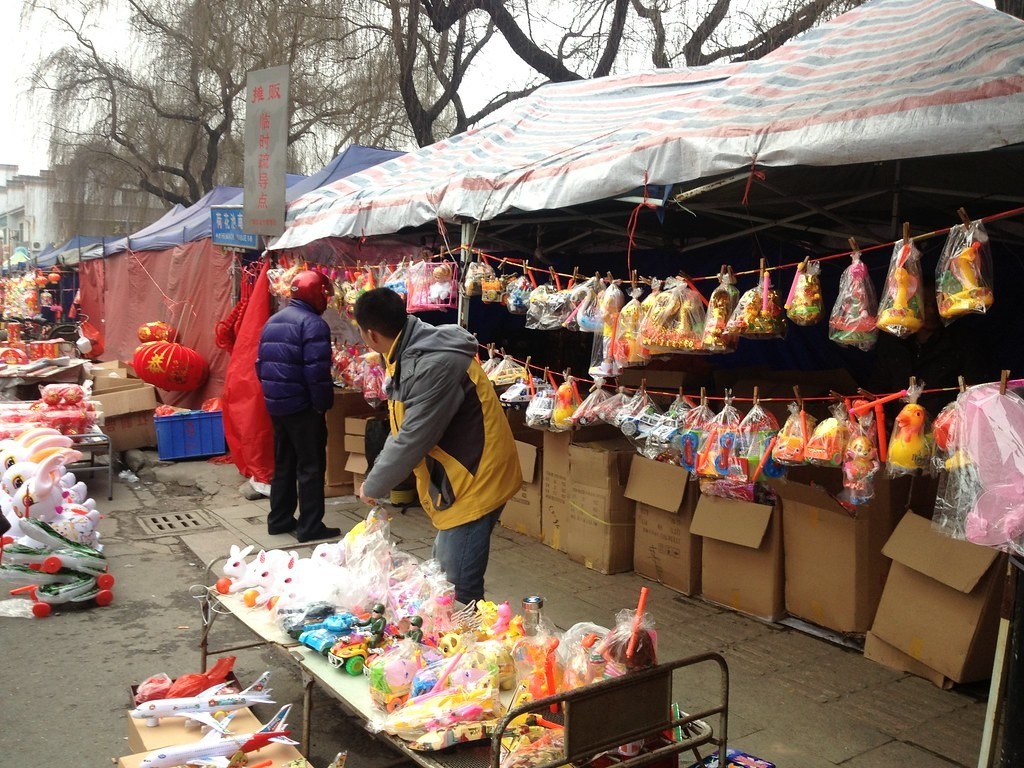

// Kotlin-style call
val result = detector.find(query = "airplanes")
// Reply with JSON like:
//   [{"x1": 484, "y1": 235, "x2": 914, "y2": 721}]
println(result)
[
  {"x1": 140, "y1": 703, "x2": 301, "y2": 768},
  {"x1": 131, "y1": 671, "x2": 277, "y2": 735},
  {"x1": 386, "y1": 675, "x2": 608, "y2": 768}
]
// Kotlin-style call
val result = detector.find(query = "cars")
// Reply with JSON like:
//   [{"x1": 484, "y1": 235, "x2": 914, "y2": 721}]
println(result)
[
  {"x1": 771, "y1": 413, "x2": 816, "y2": 466},
  {"x1": 593, "y1": 402, "x2": 639, "y2": 427},
  {"x1": 573, "y1": 403, "x2": 607, "y2": 426},
  {"x1": 806, "y1": 417, "x2": 852, "y2": 468}
]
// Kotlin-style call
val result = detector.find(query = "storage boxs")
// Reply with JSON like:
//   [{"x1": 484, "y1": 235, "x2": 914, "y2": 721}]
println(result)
[{"x1": 0, "y1": 339, "x2": 1017, "y2": 692}]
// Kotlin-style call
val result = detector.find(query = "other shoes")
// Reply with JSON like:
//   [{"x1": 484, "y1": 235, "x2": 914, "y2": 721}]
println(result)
[
  {"x1": 269, "y1": 520, "x2": 298, "y2": 534},
  {"x1": 299, "y1": 522, "x2": 341, "y2": 543}
]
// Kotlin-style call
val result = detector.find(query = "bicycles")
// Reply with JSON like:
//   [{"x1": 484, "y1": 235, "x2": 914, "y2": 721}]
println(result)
[{"x1": 0, "y1": 313, "x2": 90, "y2": 360}]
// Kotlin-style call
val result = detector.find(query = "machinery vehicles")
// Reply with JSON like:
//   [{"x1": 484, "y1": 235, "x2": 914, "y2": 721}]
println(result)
[
  {"x1": 481, "y1": 275, "x2": 532, "y2": 314},
  {"x1": 277, "y1": 601, "x2": 349, "y2": 640},
  {"x1": 363, "y1": 652, "x2": 445, "y2": 713},
  {"x1": 298, "y1": 612, "x2": 374, "y2": 656}
]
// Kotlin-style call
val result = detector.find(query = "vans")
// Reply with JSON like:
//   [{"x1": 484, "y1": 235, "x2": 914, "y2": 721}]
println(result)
[
  {"x1": 487, "y1": 367, "x2": 528, "y2": 387},
  {"x1": 500, "y1": 378, "x2": 552, "y2": 411},
  {"x1": 482, "y1": 363, "x2": 499, "y2": 377},
  {"x1": 526, "y1": 391, "x2": 556, "y2": 426}
]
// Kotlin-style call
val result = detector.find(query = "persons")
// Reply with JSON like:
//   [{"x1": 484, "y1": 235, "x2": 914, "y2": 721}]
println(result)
[
  {"x1": 353, "y1": 286, "x2": 522, "y2": 612},
  {"x1": 255, "y1": 270, "x2": 341, "y2": 543}
]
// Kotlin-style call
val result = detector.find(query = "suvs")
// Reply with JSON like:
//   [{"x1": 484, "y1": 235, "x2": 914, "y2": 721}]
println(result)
[
  {"x1": 328, "y1": 626, "x2": 406, "y2": 676},
  {"x1": 649, "y1": 419, "x2": 683, "y2": 448},
  {"x1": 621, "y1": 405, "x2": 659, "y2": 436}
]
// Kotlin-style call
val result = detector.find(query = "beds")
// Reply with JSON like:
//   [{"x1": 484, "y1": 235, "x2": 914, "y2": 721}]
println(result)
[{"x1": 200, "y1": 539, "x2": 731, "y2": 768}]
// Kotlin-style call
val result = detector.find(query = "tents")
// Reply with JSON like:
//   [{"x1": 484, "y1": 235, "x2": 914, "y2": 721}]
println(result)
[{"x1": 0, "y1": 1, "x2": 1023, "y2": 411}]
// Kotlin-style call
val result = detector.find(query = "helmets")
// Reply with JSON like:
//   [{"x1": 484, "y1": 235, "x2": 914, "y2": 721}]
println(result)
[{"x1": 290, "y1": 270, "x2": 334, "y2": 313}]
